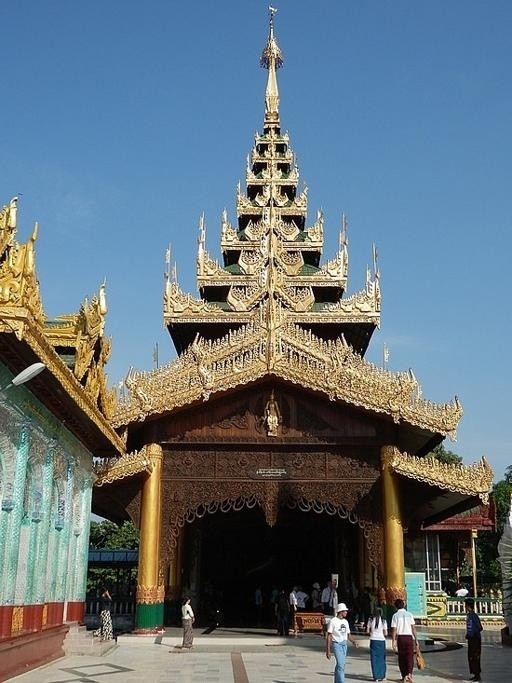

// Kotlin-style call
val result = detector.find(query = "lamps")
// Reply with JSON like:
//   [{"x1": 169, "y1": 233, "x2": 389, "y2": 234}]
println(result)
[{"x1": 2, "y1": 362, "x2": 46, "y2": 394}]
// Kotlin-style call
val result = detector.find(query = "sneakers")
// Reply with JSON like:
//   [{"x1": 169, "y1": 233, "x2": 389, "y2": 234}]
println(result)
[
  {"x1": 400, "y1": 677, "x2": 414, "y2": 683},
  {"x1": 469, "y1": 677, "x2": 481, "y2": 683}
]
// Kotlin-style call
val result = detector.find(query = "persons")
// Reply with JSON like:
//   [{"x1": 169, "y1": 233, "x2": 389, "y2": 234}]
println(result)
[
  {"x1": 181, "y1": 595, "x2": 195, "y2": 648},
  {"x1": 464, "y1": 599, "x2": 483, "y2": 681},
  {"x1": 326, "y1": 603, "x2": 359, "y2": 683},
  {"x1": 265, "y1": 392, "x2": 280, "y2": 432},
  {"x1": 366, "y1": 606, "x2": 388, "y2": 682},
  {"x1": 391, "y1": 599, "x2": 419, "y2": 683},
  {"x1": 94, "y1": 587, "x2": 113, "y2": 641},
  {"x1": 255, "y1": 580, "x2": 371, "y2": 636}
]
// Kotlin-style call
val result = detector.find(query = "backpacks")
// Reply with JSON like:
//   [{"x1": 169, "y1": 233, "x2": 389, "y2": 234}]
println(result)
[{"x1": 314, "y1": 589, "x2": 322, "y2": 603}]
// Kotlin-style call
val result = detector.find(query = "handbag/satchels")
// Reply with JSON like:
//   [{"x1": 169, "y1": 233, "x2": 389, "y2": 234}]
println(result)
[
  {"x1": 416, "y1": 653, "x2": 425, "y2": 670},
  {"x1": 320, "y1": 601, "x2": 330, "y2": 614}
]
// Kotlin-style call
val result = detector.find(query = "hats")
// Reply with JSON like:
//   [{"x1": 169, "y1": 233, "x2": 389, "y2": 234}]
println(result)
[
  {"x1": 336, "y1": 603, "x2": 349, "y2": 612},
  {"x1": 312, "y1": 583, "x2": 321, "y2": 588}
]
